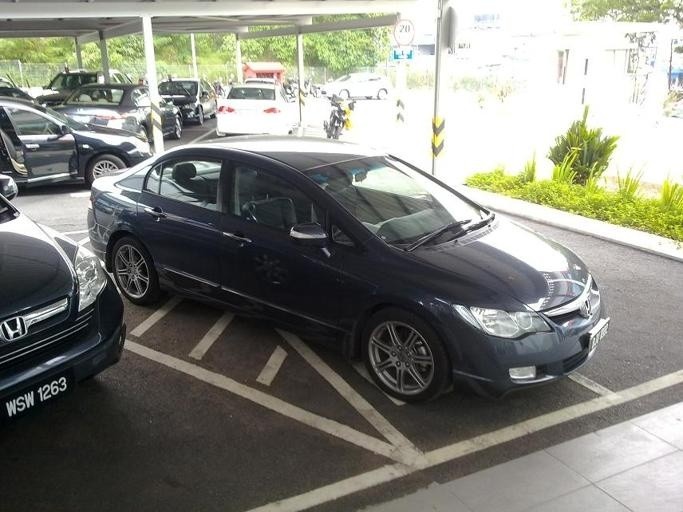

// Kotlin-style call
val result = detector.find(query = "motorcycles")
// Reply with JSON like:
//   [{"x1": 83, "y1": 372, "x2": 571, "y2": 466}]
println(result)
[{"x1": 319, "y1": 89, "x2": 356, "y2": 141}]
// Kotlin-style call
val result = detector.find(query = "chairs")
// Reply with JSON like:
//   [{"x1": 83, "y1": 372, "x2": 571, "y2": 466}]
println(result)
[
  {"x1": 313, "y1": 170, "x2": 359, "y2": 228},
  {"x1": 157, "y1": 162, "x2": 208, "y2": 208},
  {"x1": 242, "y1": 174, "x2": 298, "y2": 230}
]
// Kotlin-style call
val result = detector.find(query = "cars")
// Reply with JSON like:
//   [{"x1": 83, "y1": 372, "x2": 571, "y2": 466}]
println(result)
[
  {"x1": 1, "y1": 179, "x2": 130, "y2": 449},
  {"x1": 82, "y1": 132, "x2": 612, "y2": 410},
  {"x1": 0, "y1": 92, "x2": 153, "y2": 186}
]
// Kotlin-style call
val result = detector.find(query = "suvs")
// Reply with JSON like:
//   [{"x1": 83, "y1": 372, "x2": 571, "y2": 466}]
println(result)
[{"x1": 315, "y1": 70, "x2": 390, "y2": 102}]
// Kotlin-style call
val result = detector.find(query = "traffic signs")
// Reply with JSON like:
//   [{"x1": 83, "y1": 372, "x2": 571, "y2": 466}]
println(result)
[
  {"x1": 393, "y1": 48, "x2": 414, "y2": 60},
  {"x1": 393, "y1": 18, "x2": 414, "y2": 46}
]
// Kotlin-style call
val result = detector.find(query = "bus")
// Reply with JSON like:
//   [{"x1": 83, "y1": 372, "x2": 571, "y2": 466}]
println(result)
[{"x1": 0, "y1": 173, "x2": 18, "y2": 202}]
